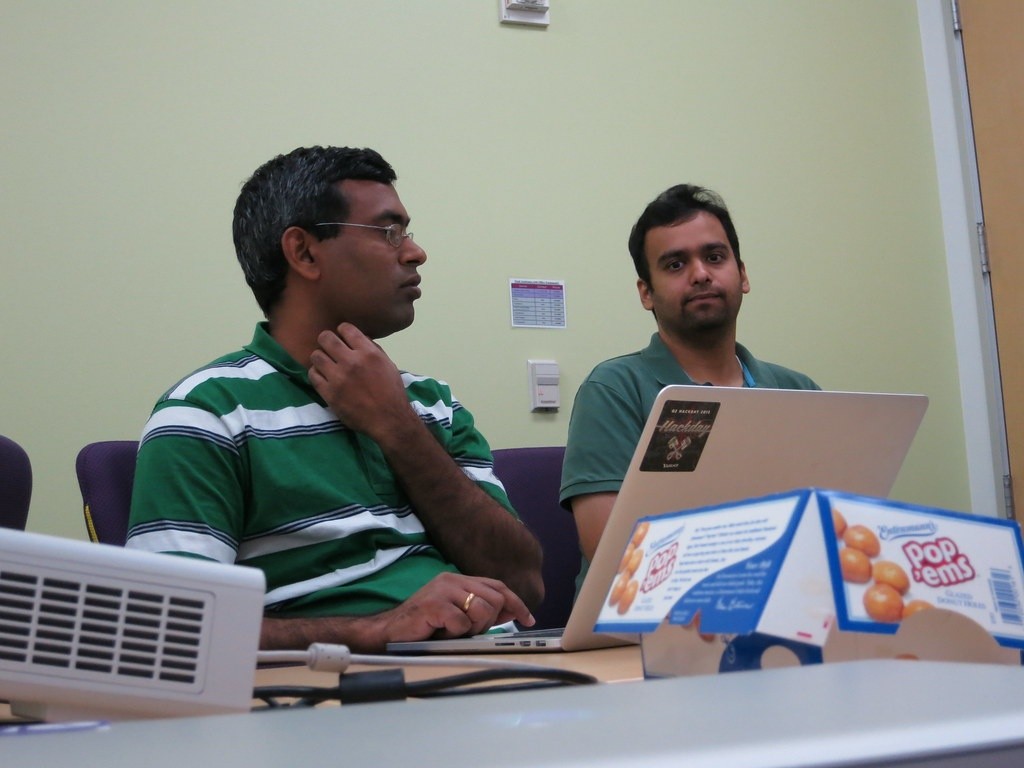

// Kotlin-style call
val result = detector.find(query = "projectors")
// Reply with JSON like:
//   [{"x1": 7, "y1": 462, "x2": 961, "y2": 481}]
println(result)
[{"x1": 0, "y1": 527, "x2": 266, "y2": 724}]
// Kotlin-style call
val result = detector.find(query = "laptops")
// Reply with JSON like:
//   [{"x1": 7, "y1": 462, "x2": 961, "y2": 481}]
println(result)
[{"x1": 385, "y1": 384, "x2": 930, "y2": 654}]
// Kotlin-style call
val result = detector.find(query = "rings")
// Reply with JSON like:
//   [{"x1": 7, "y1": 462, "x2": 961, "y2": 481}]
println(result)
[{"x1": 461, "y1": 592, "x2": 476, "y2": 612}]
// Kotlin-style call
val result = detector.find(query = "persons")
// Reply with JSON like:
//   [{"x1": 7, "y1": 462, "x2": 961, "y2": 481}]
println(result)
[
  {"x1": 558, "y1": 183, "x2": 828, "y2": 605},
  {"x1": 123, "y1": 144, "x2": 546, "y2": 649}
]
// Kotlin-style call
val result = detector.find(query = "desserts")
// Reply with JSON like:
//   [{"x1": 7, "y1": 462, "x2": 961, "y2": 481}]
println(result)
[
  {"x1": 829, "y1": 509, "x2": 944, "y2": 626},
  {"x1": 609, "y1": 519, "x2": 648, "y2": 614}
]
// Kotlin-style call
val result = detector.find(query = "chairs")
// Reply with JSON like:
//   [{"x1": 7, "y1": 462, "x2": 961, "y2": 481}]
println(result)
[
  {"x1": 493, "y1": 447, "x2": 582, "y2": 631},
  {"x1": 0, "y1": 434, "x2": 33, "y2": 531},
  {"x1": 75, "y1": 440, "x2": 138, "y2": 550}
]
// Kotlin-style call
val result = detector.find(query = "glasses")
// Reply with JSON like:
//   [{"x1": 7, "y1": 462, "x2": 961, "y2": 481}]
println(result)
[{"x1": 310, "y1": 222, "x2": 414, "y2": 249}]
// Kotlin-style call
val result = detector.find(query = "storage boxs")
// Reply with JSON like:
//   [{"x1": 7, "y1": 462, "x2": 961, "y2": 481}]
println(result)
[{"x1": 591, "y1": 486, "x2": 1024, "y2": 680}]
